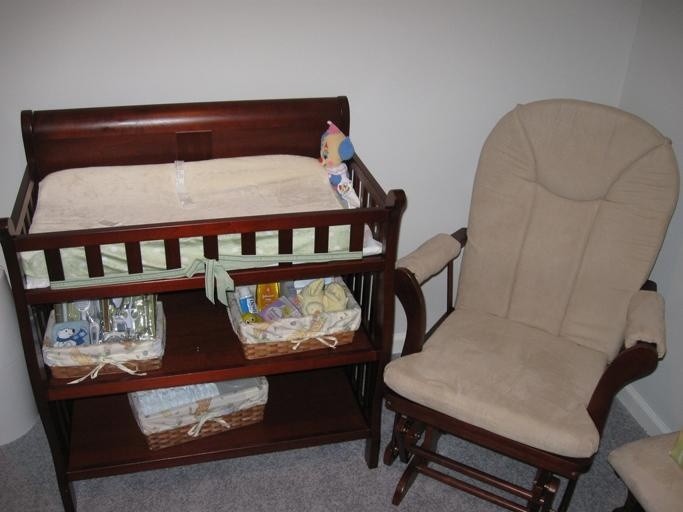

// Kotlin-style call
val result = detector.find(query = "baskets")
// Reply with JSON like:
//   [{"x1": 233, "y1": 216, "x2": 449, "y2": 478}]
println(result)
[
  {"x1": 127, "y1": 376, "x2": 269, "y2": 452},
  {"x1": 226, "y1": 277, "x2": 362, "y2": 361},
  {"x1": 42, "y1": 300, "x2": 166, "y2": 380}
]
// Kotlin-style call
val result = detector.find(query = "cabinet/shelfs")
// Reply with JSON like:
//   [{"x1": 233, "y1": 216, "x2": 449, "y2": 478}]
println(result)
[{"x1": 0, "y1": 95, "x2": 408, "y2": 512}]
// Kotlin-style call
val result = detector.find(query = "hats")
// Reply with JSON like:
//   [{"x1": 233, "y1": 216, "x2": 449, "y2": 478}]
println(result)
[{"x1": 324, "y1": 121, "x2": 341, "y2": 136}]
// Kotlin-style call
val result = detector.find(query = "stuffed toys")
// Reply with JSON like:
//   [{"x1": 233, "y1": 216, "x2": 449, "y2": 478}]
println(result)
[{"x1": 318, "y1": 123, "x2": 354, "y2": 192}]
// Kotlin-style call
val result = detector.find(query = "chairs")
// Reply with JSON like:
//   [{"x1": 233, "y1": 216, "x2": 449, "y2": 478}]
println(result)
[{"x1": 382, "y1": 98, "x2": 681, "y2": 512}]
